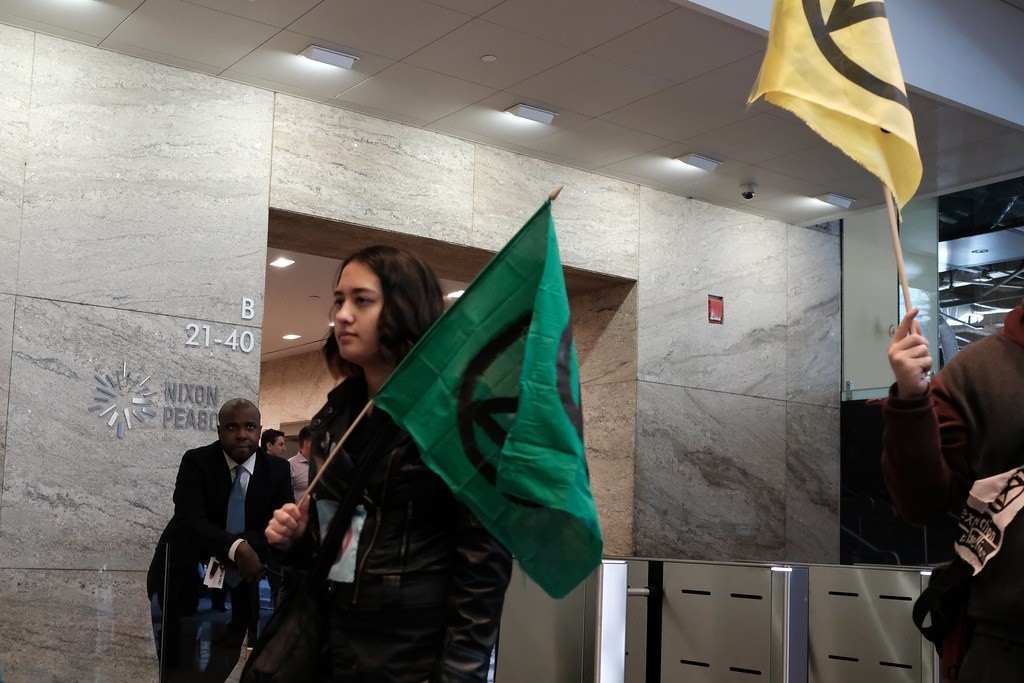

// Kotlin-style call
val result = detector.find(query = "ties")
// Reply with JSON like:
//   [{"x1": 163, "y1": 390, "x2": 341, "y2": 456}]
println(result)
[{"x1": 223, "y1": 466, "x2": 246, "y2": 590}]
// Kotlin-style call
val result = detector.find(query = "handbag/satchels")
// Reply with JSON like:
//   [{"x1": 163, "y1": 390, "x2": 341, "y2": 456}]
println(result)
[
  {"x1": 913, "y1": 563, "x2": 981, "y2": 683},
  {"x1": 239, "y1": 596, "x2": 328, "y2": 683}
]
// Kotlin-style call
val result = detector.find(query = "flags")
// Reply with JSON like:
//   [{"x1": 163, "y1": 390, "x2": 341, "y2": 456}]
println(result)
[
  {"x1": 748, "y1": 0, "x2": 923, "y2": 222},
  {"x1": 374, "y1": 201, "x2": 604, "y2": 598}
]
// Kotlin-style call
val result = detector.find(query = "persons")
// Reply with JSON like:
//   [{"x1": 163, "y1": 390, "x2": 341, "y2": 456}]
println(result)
[
  {"x1": 877, "y1": 301, "x2": 1024, "y2": 683},
  {"x1": 146, "y1": 398, "x2": 294, "y2": 683},
  {"x1": 286, "y1": 425, "x2": 310, "y2": 504},
  {"x1": 241, "y1": 244, "x2": 514, "y2": 683},
  {"x1": 261, "y1": 428, "x2": 286, "y2": 457}
]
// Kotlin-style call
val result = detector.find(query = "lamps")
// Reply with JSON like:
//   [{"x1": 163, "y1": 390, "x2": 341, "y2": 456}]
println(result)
[
  {"x1": 296, "y1": 44, "x2": 360, "y2": 69},
  {"x1": 671, "y1": 153, "x2": 722, "y2": 172},
  {"x1": 816, "y1": 192, "x2": 855, "y2": 209},
  {"x1": 503, "y1": 103, "x2": 558, "y2": 126}
]
